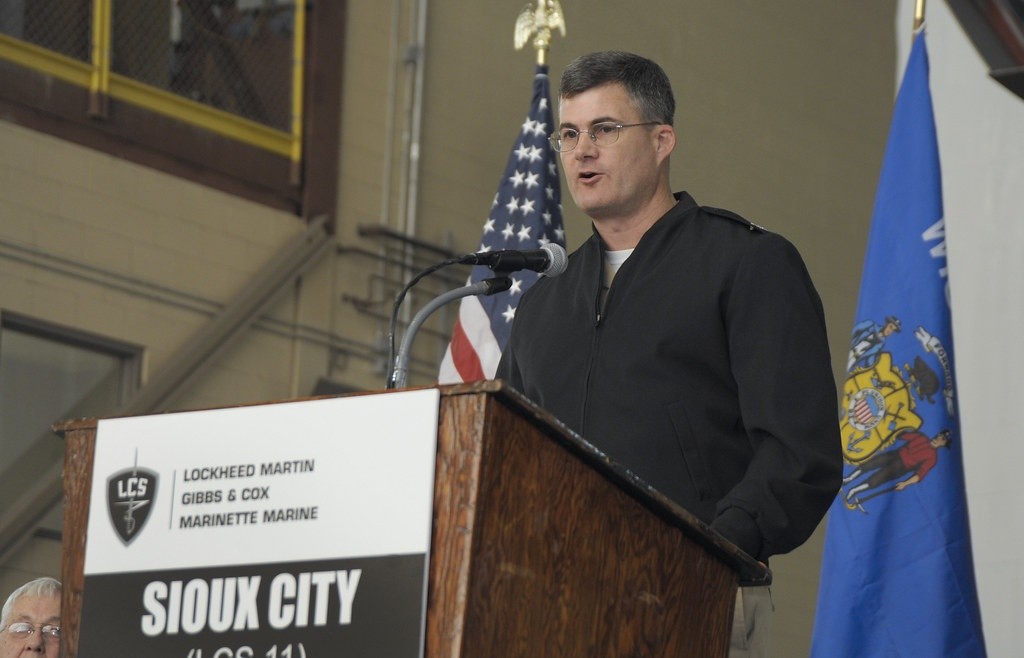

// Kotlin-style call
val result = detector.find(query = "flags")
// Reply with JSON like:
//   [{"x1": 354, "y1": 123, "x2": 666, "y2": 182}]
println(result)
[
  {"x1": 437, "y1": 64, "x2": 567, "y2": 385},
  {"x1": 809, "y1": 27, "x2": 987, "y2": 658}
]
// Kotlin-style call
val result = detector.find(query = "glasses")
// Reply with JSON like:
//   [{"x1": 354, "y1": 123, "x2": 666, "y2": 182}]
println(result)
[
  {"x1": 0, "y1": 621, "x2": 62, "y2": 642},
  {"x1": 548, "y1": 120, "x2": 662, "y2": 153}
]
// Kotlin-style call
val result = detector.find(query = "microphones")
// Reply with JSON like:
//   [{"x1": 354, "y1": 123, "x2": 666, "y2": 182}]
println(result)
[{"x1": 466, "y1": 243, "x2": 567, "y2": 277}]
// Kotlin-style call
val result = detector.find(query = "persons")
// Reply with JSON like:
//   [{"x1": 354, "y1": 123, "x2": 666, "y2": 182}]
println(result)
[
  {"x1": 493, "y1": 50, "x2": 844, "y2": 562},
  {"x1": 0, "y1": 576, "x2": 62, "y2": 658}
]
